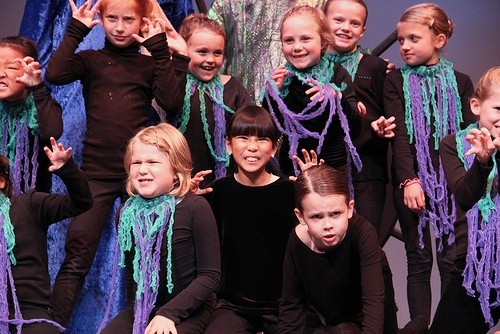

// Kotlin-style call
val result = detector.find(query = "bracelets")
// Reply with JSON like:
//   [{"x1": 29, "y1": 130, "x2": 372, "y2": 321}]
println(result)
[{"x1": 403, "y1": 181, "x2": 422, "y2": 186}]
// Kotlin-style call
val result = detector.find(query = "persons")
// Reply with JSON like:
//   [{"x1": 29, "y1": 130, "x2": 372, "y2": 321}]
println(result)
[{"x1": 0, "y1": 0, "x2": 500, "y2": 334}]
